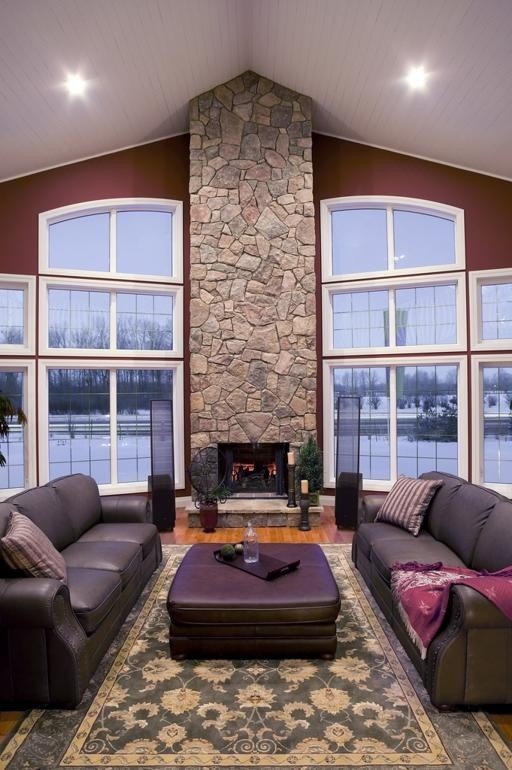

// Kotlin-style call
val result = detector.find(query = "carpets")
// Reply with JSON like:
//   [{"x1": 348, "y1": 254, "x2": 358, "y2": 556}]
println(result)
[{"x1": 1, "y1": 540, "x2": 512, "y2": 770}]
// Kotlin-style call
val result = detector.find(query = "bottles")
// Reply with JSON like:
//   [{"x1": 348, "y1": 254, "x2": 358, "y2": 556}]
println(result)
[{"x1": 243, "y1": 520, "x2": 259, "y2": 564}]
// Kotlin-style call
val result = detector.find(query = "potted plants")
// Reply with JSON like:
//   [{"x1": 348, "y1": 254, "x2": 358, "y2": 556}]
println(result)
[
  {"x1": 220, "y1": 544, "x2": 236, "y2": 562},
  {"x1": 192, "y1": 483, "x2": 233, "y2": 535}
]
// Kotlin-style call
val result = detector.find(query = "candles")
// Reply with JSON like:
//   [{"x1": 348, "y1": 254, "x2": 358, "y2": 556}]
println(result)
[
  {"x1": 287, "y1": 452, "x2": 295, "y2": 465},
  {"x1": 300, "y1": 479, "x2": 309, "y2": 494}
]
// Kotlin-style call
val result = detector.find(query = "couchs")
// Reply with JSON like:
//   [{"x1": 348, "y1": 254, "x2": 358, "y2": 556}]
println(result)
[
  {"x1": 1, "y1": 472, "x2": 164, "y2": 710},
  {"x1": 350, "y1": 471, "x2": 512, "y2": 714}
]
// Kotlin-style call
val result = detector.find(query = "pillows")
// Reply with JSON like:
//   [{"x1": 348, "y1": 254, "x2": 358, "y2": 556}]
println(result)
[
  {"x1": 2, "y1": 510, "x2": 68, "y2": 588},
  {"x1": 373, "y1": 474, "x2": 444, "y2": 536}
]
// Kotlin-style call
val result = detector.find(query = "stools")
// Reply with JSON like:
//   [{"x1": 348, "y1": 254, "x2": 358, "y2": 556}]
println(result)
[{"x1": 167, "y1": 542, "x2": 341, "y2": 662}]
